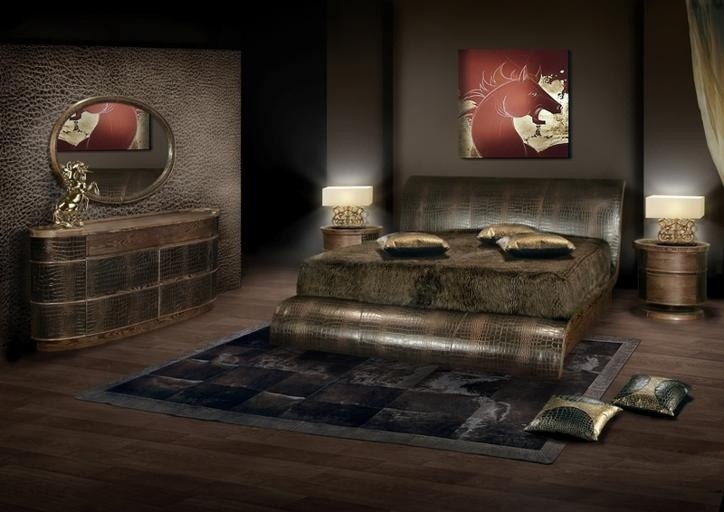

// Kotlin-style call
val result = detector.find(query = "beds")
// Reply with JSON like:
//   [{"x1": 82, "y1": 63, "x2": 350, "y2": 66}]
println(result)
[{"x1": 270, "y1": 175, "x2": 625, "y2": 380}]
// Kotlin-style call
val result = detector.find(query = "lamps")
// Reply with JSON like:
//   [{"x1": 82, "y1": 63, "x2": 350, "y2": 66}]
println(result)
[
  {"x1": 322, "y1": 185, "x2": 373, "y2": 229},
  {"x1": 646, "y1": 195, "x2": 706, "y2": 246}
]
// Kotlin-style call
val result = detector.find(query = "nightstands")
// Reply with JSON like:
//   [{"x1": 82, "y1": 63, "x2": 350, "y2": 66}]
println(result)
[
  {"x1": 321, "y1": 226, "x2": 383, "y2": 252},
  {"x1": 634, "y1": 239, "x2": 710, "y2": 321}
]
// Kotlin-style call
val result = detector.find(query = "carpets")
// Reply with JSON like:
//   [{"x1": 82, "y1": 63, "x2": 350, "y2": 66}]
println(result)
[{"x1": 73, "y1": 314, "x2": 642, "y2": 464}]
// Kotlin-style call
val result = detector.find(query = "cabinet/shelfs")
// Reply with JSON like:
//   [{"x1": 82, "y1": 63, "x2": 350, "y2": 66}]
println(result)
[{"x1": 24, "y1": 207, "x2": 222, "y2": 352}]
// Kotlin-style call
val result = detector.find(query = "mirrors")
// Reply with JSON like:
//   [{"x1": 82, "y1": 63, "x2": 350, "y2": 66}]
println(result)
[{"x1": 50, "y1": 95, "x2": 174, "y2": 205}]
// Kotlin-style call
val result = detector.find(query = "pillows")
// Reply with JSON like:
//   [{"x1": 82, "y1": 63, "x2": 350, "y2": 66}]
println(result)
[
  {"x1": 477, "y1": 224, "x2": 539, "y2": 244},
  {"x1": 377, "y1": 231, "x2": 450, "y2": 257},
  {"x1": 612, "y1": 373, "x2": 691, "y2": 417},
  {"x1": 523, "y1": 392, "x2": 623, "y2": 442},
  {"x1": 495, "y1": 232, "x2": 576, "y2": 258}
]
